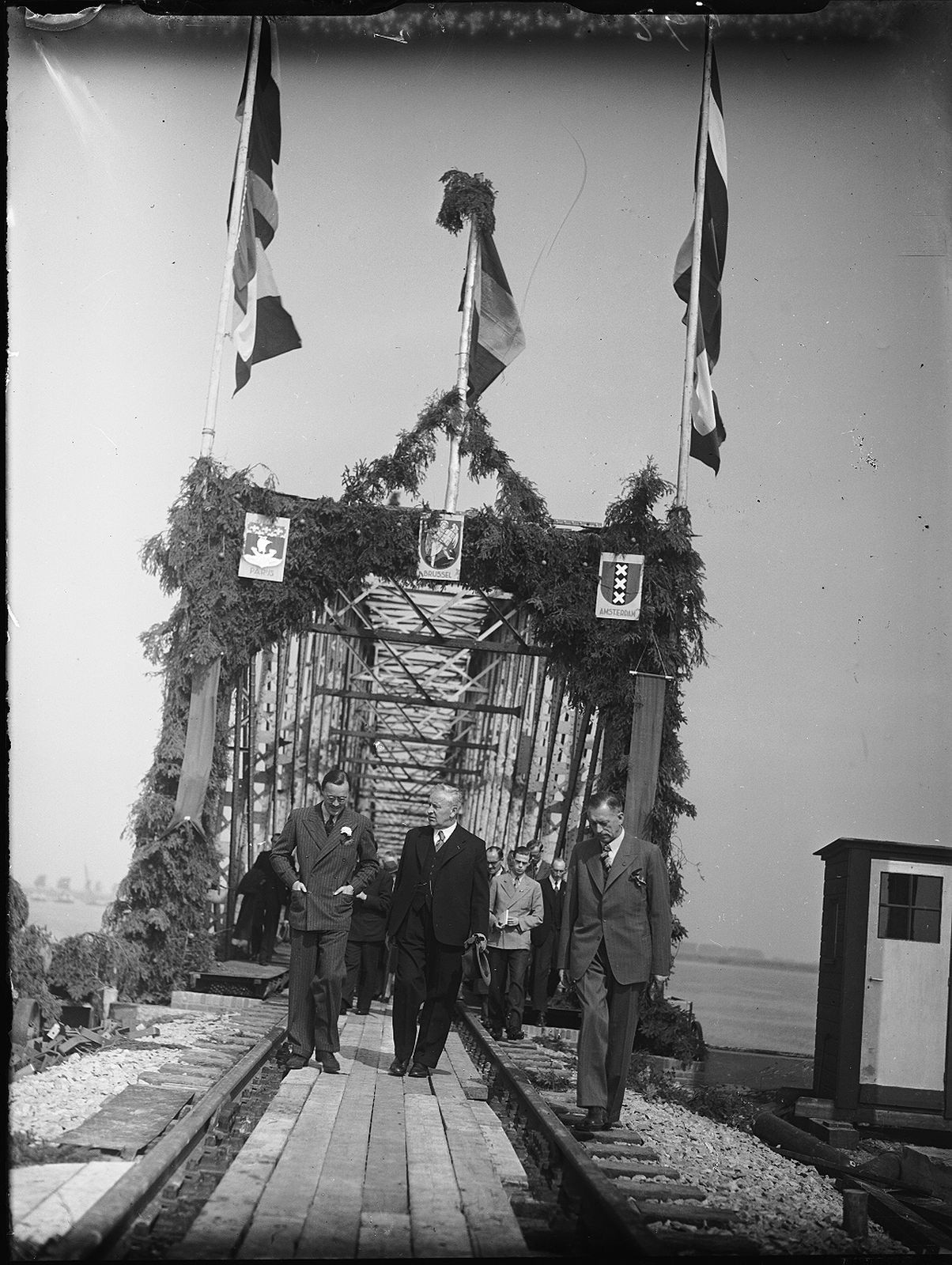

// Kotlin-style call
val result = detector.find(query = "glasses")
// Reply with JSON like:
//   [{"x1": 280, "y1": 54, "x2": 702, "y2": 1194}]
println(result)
[
  {"x1": 487, "y1": 857, "x2": 501, "y2": 865},
  {"x1": 328, "y1": 795, "x2": 347, "y2": 802}
]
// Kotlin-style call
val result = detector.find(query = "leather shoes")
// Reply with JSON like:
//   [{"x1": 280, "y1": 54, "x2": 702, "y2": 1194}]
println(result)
[
  {"x1": 408, "y1": 1062, "x2": 430, "y2": 1078},
  {"x1": 390, "y1": 1056, "x2": 409, "y2": 1076}
]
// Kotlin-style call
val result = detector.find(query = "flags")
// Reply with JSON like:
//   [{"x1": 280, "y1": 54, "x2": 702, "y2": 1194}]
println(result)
[
  {"x1": 225, "y1": 14, "x2": 303, "y2": 399},
  {"x1": 673, "y1": 31, "x2": 728, "y2": 478},
  {"x1": 458, "y1": 213, "x2": 526, "y2": 404}
]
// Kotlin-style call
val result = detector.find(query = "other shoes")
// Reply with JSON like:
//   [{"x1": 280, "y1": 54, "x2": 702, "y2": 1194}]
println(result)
[
  {"x1": 490, "y1": 1030, "x2": 502, "y2": 1040},
  {"x1": 355, "y1": 1009, "x2": 367, "y2": 1015},
  {"x1": 380, "y1": 997, "x2": 389, "y2": 1004},
  {"x1": 586, "y1": 1113, "x2": 603, "y2": 1131},
  {"x1": 351, "y1": 1008, "x2": 359, "y2": 1014},
  {"x1": 251, "y1": 954, "x2": 257, "y2": 961},
  {"x1": 259, "y1": 961, "x2": 269, "y2": 966},
  {"x1": 340, "y1": 1008, "x2": 346, "y2": 1015},
  {"x1": 536, "y1": 1012, "x2": 546, "y2": 1027},
  {"x1": 574, "y1": 1116, "x2": 610, "y2": 1141},
  {"x1": 478, "y1": 1020, "x2": 491, "y2": 1032},
  {"x1": 508, "y1": 1031, "x2": 524, "y2": 1040}
]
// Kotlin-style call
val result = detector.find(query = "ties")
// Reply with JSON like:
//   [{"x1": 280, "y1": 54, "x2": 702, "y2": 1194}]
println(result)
[
  {"x1": 489, "y1": 873, "x2": 496, "y2": 882},
  {"x1": 435, "y1": 831, "x2": 445, "y2": 853},
  {"x1": 532, "y1": 864, "x2": 537, "y2": 880},
  {"x1": 325, "y1": 815, "x2": 335, "y2": 837},
  {"x1": 554, "y1": 881, "x2": 559, "y2": 894},
  {"x1": 513, "y1": 876, "x2": 520, "y2": 892},
  {"x1": 599, "y1": 845, "x2": 611, "y2": 874}
]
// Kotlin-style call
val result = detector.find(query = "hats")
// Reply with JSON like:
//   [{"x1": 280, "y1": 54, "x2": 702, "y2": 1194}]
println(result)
[{"x1": 461, "y1": 943, "x2": 491, "y2": 987}]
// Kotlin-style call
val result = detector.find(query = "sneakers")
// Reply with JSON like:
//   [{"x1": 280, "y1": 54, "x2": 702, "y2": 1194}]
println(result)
[
  {"x1": 285, "y1": 1053, "x2": 308, "y2": 1069},
  {"x1": 315, "y1": 1049, "x2": 341, "y2": 1073}
]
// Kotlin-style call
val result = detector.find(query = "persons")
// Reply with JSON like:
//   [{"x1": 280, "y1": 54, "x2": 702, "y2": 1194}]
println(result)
[
  {"x1": 470, "y1": 841, "x2": 551, "y2": 1038},
  {"x1": 339, "y1": 857, "x2": 401, "y2": 1015},
  {"x1": 557, "y1": 791, "x2": 670, "y2": 1131},
  {"x1": 270, "y1": 771, "x2": 380, "y2": 1072},
  {"x1": 531, "y1": 858, "x2": 565, "y2": 1025},
  {"x1": 388, "y1": 784, "x2": 490, "y2": 1078},
  {"x1": 232, "y1": 833, "x2": 296, "y2": 965}
]
